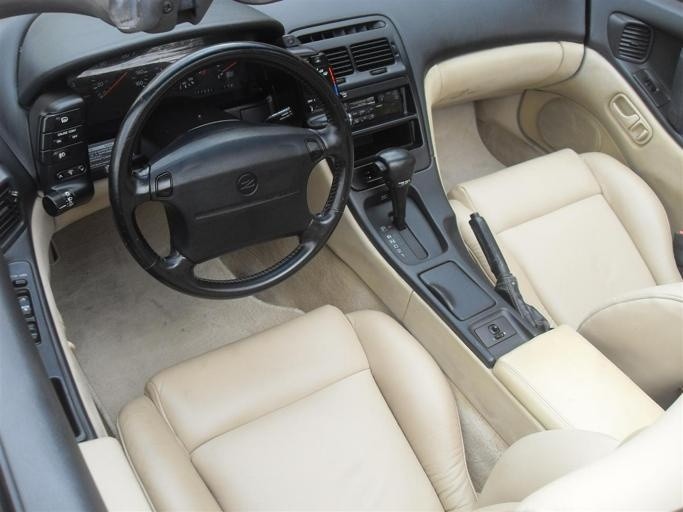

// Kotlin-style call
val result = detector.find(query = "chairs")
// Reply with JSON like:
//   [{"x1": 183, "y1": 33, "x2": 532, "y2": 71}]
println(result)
[
  {"x1": 446, "y1": 147, "x2": 683, "y2": 410},
  {"x1": 116, "y1": 300, "x2": 683, "y2": 512}
]
